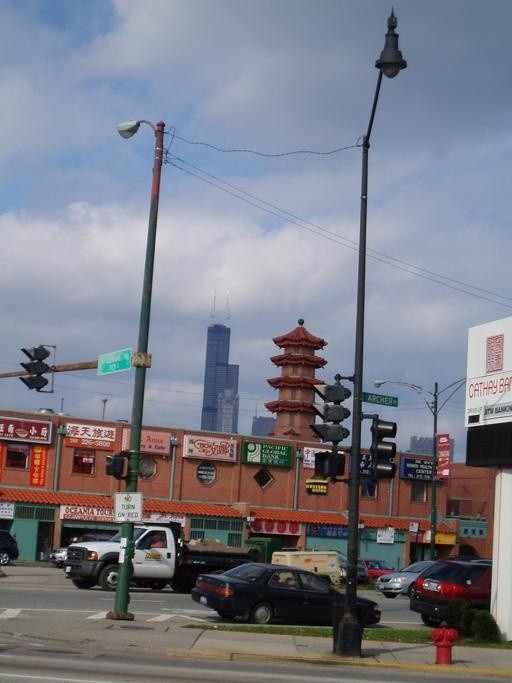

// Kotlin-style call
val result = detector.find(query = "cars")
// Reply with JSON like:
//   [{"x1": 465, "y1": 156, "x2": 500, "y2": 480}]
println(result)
[
  {"x1": 190, "y1": 561, "x2": 383, "y2": 628},
  {"x1": 271, "y1": 545, "x2": 492, "y2": 630}
]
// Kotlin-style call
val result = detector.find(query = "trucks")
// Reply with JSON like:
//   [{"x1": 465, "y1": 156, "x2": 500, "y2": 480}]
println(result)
[{"x1": 62, "y1": 522, "x2": 266, "y2": 595}]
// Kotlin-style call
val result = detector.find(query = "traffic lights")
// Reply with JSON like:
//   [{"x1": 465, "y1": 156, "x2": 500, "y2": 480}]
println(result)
[
  {"x1": 105, "y1": 453, "x2": 125, "y2": 478},
  {"x1": 369, "y1": 416, "x2": 399, "y2": 482},
  {"x1": 18, "y1": 344, "x2": 50, "y2": 391},
  {"x1": 312, "y1": 451, "x2": 346, "y2": 479},
  {"x1": 309, "y1": 382, "x2": 349, "y2": 443}
]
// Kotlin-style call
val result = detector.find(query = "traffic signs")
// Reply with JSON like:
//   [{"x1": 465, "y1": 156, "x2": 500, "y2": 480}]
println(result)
[
  {"x1": 362, "y1": 391, "x2": 398, "y2": 407},
  {"x1": 96, "y1": 349, "x2": 132, "y2": 376},
  {"x1": 112, "y1": 490, "x2": 143, "y2": 525}
]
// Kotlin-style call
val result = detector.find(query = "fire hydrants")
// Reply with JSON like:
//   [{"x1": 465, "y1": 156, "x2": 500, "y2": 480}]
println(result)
[{"x1": 429, "y1": 626, "x2": 459, "y2": 666}]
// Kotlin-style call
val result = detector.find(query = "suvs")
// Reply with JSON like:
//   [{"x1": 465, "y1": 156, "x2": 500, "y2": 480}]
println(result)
[
  {"x1": 49, "y1": 534, "x2": 111, "y2": 569},
  {"x1": 1, "y1": 528, "x2": 19, "y2": 567}
]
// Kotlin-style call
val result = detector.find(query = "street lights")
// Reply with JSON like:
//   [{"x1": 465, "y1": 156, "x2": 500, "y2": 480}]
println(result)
[
  {"x1": 330, "y1": 6, "x2": 409, "y2": 661},
  {"x1": 373, "y1": 376, "x2": 468, "y2": 563},
  {"x1": 106, "y1": 113, "x2": 166, "y2": 623},
  {"x1": 101, "y1": 396, "x2": 108, "y2": 419}
]
